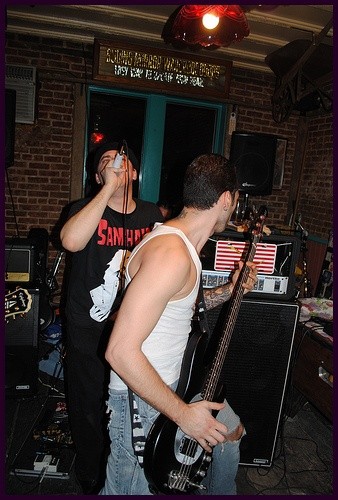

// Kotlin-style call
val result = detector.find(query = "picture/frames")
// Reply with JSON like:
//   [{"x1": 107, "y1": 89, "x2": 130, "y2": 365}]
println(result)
[{"x1": 272, "y1": 137, "x2": 289, "y2": 192}]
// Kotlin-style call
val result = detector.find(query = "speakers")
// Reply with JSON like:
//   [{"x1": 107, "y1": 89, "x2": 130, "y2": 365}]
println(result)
[
  {"x1": 227, "y1": 130, "x2": 278, "y2": 196},
  {"x1": 5, "y1": 88, "x2": 17, "y2": 168},
  {"x1": 5, "y1": 290, "x2": 41, "y2": 396},
  {"x1": 195, "y1": 295, "x2": 302, "y2": 468}
]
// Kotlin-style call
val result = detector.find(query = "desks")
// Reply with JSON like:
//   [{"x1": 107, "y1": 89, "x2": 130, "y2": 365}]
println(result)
[{"x1": 286, "y1": 321, "x2": 334, "y2": 425}]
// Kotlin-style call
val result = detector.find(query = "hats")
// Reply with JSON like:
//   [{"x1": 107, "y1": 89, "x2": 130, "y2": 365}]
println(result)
[{"x1": 93, "y1": 143, "x2": 134, "y2": 173}]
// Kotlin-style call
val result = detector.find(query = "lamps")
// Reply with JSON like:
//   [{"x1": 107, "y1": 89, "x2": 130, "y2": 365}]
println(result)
[{"x1": 171, "y1": 5, "x2": 250, "y2": 47}]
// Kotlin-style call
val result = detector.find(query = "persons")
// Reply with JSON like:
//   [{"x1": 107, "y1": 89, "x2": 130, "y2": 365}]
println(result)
[
  {"x1": 158, "y1": 201, "x2": 177, "y2": 219},
  {"x1": 50, "y1": 141, "x2": 165, "y2": 496},
  {"x1": 98, "y1": 153, "x2": 257, "y2": 495}
]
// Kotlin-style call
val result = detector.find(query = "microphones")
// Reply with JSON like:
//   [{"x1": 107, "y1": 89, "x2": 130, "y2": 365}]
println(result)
[{"x1": 113, "y1": 146, "x2": 126, "y2": 173}]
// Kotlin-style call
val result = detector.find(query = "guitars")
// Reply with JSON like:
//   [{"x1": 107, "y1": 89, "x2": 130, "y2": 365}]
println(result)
[{"x1": 140, "y1": 203, "x2": 272, "y2": 497}]
